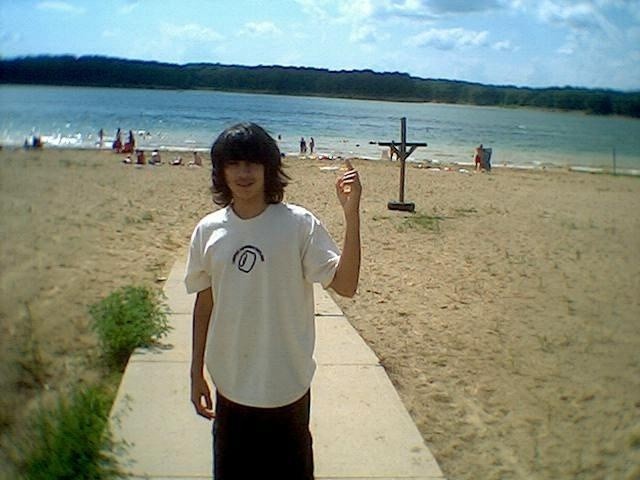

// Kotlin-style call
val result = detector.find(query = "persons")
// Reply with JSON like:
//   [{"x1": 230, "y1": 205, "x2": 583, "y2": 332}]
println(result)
[
  {"x1": 115, "y1": 128, "x2": 123, "y2": 153},
  {"x1": 185, "y1": 122, "x2": 363, "y2": 479},
  {"x1": 98, "y1": 128, "x2": 103, "y2": 143},
  {"x1": 187, "y1": 151, "x2": 201, "y2": 167},
  {"x1": 123, "y1": 130, "x2": 134, "y2": 153},
  {"x1": 474, "y1": 144, "x2": 484, "y2": 171},
  {"x1": 310, "y1": 137, "x2": 314, "y2": 153},
  {"x1": 300, "y1": 137, "x2": 306, "y2": 153},
  {"x1": 147, "y1": 149, "x2": 161, "y2": 165},
  {"x1": 134, "y1": 149, "x2": 147, "y2": 165}
]
